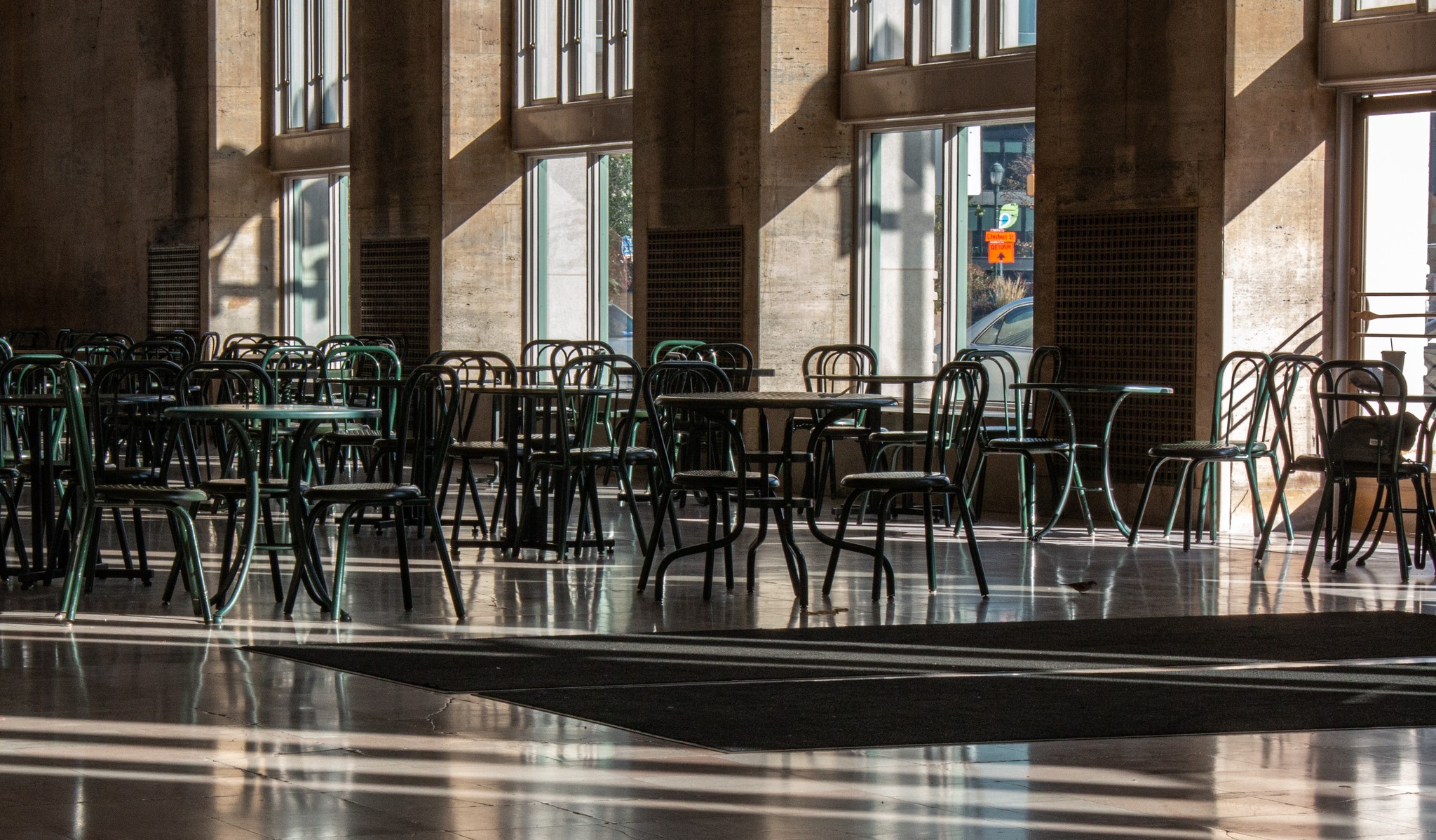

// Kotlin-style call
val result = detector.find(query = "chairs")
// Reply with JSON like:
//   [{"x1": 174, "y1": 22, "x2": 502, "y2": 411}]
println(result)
[{"x1": 2, "y1": 333, "x2": 1436, "y2": 631}]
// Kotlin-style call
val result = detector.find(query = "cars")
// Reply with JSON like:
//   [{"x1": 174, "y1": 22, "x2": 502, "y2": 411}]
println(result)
[{"x1": 933, "y1": 296, "x2": 1033, "y2": 404}]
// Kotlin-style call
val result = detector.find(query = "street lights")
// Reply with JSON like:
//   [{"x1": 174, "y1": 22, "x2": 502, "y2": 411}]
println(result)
[{"x1": 989, "y1": 161, "x2": 1005, "y2": 310}]
